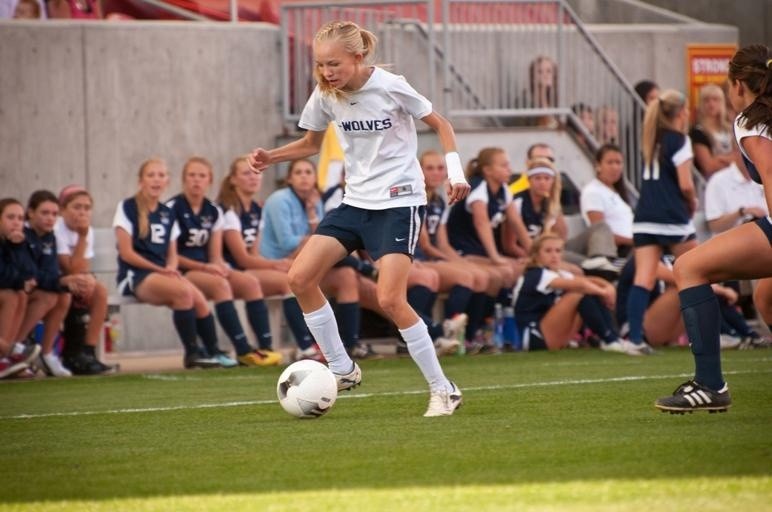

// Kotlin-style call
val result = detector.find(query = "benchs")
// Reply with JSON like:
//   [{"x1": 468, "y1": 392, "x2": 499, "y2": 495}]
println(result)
[{"x1": 91, "y1": 226, "x2": 298, "y2": 360}]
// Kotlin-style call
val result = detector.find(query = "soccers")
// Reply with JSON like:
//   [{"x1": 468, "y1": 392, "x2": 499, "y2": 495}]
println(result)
[{"x1": 276, "y1": 359, "x2": 338, "y2": 418}]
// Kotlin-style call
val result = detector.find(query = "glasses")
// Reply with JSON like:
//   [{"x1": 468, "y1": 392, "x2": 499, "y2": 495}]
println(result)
[{"x1": 546, "y1": 157, "x2": 555, "y2": 163}]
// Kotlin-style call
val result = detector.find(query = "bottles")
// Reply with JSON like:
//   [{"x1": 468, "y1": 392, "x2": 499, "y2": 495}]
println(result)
[
  {"x1": 485, "y1": 304, "x2": 521, "y2": 351},
  {"x1": 104, "y1": 315, "x2": 111, "y2": 352},
  {"x1": 111, "y1": 319, "x2": 123, "y2": 353}
]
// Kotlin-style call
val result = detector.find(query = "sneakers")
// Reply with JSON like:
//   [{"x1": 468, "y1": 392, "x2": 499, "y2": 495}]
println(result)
[
  {"x1": 184, "y1": 339, "x2": 369, "y2": 368},
  {"x1": 331, "y1": 361, "x2": 361, "y2": 392},
  {"x1": 653, "y1": 377, "x2": 732, "y2": 414},
  {"x1": 0, "y1": 343, "x2": 119, "y2": 378},
  {"x1": 739, "y1": 331, "x2": 771, "y2": 352},
  {"x1": 631, "y1": 342, "x2": 658, "y2": 356},
  {"x1": 434, "y1": 312, "x2": 469, "y2": 357},
  {"x1": 718, "y1": 333, "x2": 741, "y2": 350},
  {"x1": 600, "y1": 338, "x2": 632, "y2": 354},
  {"x1": 424, "y1": 383, "x2": 463, "y2": 417}
]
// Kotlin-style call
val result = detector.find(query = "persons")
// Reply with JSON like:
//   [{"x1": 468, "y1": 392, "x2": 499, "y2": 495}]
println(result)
[{"x1": 1, "y1": 21, "x2": 772, "y2": 416}]
